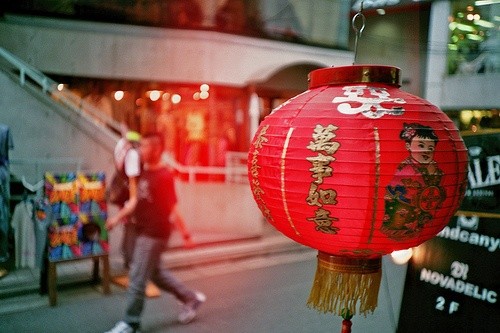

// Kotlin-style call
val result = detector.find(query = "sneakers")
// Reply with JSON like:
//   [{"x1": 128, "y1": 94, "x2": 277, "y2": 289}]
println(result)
[
  {"x1": 103, "y1": 322, "x2": 143, "y2": 332},
  {"x1": 176, "y1": 292, "x2": 206, "y2": 324}
]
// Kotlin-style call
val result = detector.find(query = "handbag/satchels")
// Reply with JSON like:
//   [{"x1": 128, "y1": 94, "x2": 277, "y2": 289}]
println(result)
[{"x1": 108, "y1": 147, "x2": 147, "y2": 209}]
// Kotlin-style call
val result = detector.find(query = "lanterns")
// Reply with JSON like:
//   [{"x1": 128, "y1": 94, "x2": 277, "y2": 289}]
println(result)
[{"x1": 246, "y1": 66, "x2": 468, "y2": 333}]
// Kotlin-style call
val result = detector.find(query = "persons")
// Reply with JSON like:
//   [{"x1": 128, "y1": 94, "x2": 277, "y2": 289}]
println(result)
[
  {"x1": 104, "y1": 135, "x2": 206, "y2": 333},
  {"x1": 106, "y1": 131, "x2": 164, "y2": 298}
]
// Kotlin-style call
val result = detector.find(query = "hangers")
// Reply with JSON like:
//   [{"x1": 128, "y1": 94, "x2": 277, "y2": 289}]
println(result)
[{"x1": 17, "y1": 188, "x2": 32, "y2": 209}]
